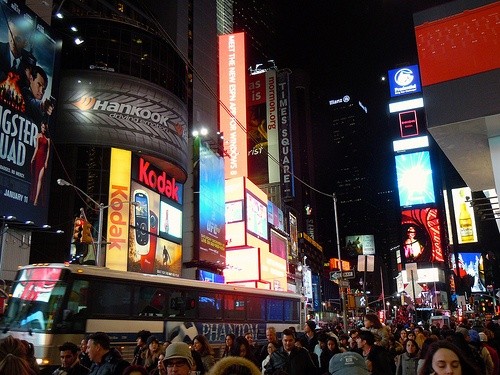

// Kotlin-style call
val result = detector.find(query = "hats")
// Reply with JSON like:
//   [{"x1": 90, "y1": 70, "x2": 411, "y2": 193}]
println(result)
[
  {"x1": 328, "y1": 352, "x2": 372, "y2": 375},
  {"x1": 162, "y1": 342, "x2": 194, "y2": 370},
  {"x1": 467, "y1": 330, "x2": 480, "y2": 343},
  {"x1": 478, "y1": 332, "x2": 488, "y2": 343}
]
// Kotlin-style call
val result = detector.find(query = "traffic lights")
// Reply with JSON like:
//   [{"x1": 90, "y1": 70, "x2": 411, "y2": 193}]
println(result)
[
  {"x1": 73, "y1": 217, "x2": 83, "y2": 239},
  {"x1": 81, "y1": 222, "x2": 92, "y2": 243}
]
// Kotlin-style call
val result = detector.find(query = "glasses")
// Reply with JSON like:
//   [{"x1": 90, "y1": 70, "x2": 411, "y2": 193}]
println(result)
[{"x1": 164, "y1": 361, "x2": 186, "y2": 368}]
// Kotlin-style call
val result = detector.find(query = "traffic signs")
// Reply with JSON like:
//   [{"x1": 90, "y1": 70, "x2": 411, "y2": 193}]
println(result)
[{"x1": 329, "y1": 269, "x2": 356, "y2": 281}]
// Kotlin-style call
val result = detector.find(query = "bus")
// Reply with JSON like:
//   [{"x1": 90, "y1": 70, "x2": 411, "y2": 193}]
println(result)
[{"x1": 0, "y1": 262, "x2": 308, "y2": 375}]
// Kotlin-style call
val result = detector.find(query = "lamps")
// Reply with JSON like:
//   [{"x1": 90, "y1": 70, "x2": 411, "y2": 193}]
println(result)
[
  {"x1": 0, "y1": 215, "x2": 64, "y2": 234},
  {"x1": 465, "y1": 195, "x2": 500, "y2": 219},
  {"x1": 56, "y1": 0, "x2": 69, "y2": 19}
]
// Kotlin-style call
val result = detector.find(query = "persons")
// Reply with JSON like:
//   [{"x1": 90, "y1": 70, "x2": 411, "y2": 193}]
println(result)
[
  {"x1": 0, "y1": 306, "x2": 500, "y2": 375},
  {"x1": 404, "y1": 226, "x2": 422, "y2": 261},
  {"x1": 0, "y1": 19, "x2": 54, "y2": 208}
]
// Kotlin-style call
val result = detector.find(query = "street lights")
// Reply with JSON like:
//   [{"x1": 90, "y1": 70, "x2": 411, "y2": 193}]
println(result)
[{"x1": 56, "y1": 178, "x2": 141, "y2": 266}]
